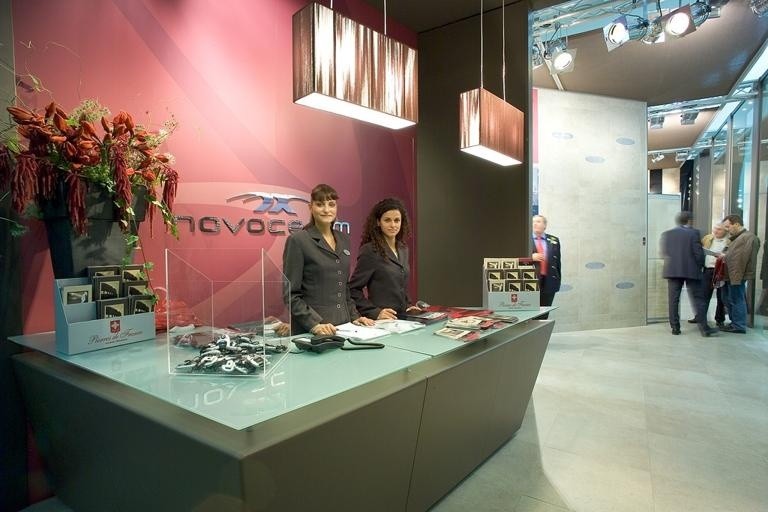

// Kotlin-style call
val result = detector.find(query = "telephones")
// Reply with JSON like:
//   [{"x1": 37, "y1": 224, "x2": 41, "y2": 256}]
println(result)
[{"x1": 293, "y1": 332, "x2": 384, "y2": 353}]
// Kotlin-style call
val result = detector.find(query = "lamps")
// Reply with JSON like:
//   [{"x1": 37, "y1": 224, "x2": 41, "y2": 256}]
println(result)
[
  {"x1": 658, "y1": 0, "x2": 709, "y2": 40},
  {"x1": 694, "y1": 0, "x2": 730, "y2": 19},
  {"x1": 602, "y1": 1, "x2": 650, "y2": 52},
  {"x1": 747, "y1": 0, "x2": 768, "y2": 19},
  {"x1": 458, "y1": 0, "x2": 525, "y2": 166},
  {"x1": 649, "y1": 115, "x2": 664, "y2": 129},
  {"x1": 651, "y1": 152, "x2": 665, "y2": 163},
  {"x1": 675, "y1": 150, "x2": 687, "y2": 162},
  {"x1": 640, "y1": 0, "x2": 665, "y2": 46},
  {"x1": 542, "y1": 21, "x2": 577, "y2": 75},
  {"x1": 681, "y1": 107, "x2": 700, "y2": 125},
  {"x1": 532, "y1": 46, "x2": 544, "y2": 71},
  {"x1": 292, "y1": 0, "x2": 419, "y2": 131}
]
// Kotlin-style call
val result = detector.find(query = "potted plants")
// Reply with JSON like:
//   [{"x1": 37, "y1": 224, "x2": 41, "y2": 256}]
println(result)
[{"x1": 0, "y1": 37, "x2": 181, "y2": 279}]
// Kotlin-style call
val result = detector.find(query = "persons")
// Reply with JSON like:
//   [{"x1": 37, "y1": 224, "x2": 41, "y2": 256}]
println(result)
[
  {"x1": 716, "y1": 215, "x2": 761, "y2": 333},
  {"x1": 282, "y1": 184, "x2": 375, "y2": 336},
  {"x1": 349, "y1": 197, "x2": 422, "y2": 320},
  {"x1": 660, "y1": 212, "x2": 719, "y2": 337},
  {"x1": 532, "y1": 215, "x2": 561, "y2": 319},
  {"x1": 688, "y1": 221, "x2": 731, "y2": 327}
]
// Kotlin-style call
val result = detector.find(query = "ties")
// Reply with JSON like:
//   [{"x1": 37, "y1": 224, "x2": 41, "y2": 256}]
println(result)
[{"x1": 537, "y1": 233, "x2": 546, "y2": 277}]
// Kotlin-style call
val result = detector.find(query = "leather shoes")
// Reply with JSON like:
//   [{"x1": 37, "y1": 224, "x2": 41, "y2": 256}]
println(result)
[
  {"x1": 672, "y1": 327, "x2": 682, "y2": 333},
  {"x1": 722, "y1": 324, "x2": 747, "y2": 334},
  {"x1": 701, "y1": 327, "x2": 718, "y2": 337},
  {"x1": 688, "y1": 316, "x2": 698, "y2": 322}
]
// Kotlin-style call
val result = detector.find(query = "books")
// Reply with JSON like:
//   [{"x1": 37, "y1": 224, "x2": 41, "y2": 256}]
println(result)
[
  {"x1": 484, "y1": 258, "x2": 534, "y2": 268},
  {"x1": 87, "y1": 264, "x2": 143, "y2": 283},
  {"x1": 61, "y1": 274, "x2": 148, "y2": 304},
  {"x1": 488, "y1": 280, "x2": 539, "y2": 292},
  {"x1": 486, "y1": 268, "x2": 537, "y2": 279},
  {"x1": 97, "y1": 295, "x2": 152, "y2": 319},
  {"x1": 227, "y1": 315, "x2": 282, "y2": 332},
  {"x1": 434, "y1": 313, "x2": 518, "y2": 340},
  {"x1": 394, "y1": 309, "x2": 447, "y2": 324},
  {"x1": 334, "y1": 320, "x2": 426, "y2": 341}
]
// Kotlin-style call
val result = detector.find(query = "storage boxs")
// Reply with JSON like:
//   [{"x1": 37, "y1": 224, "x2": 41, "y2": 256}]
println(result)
[
  {"x1": 166, "y1": 247, "x2": 293, "y2": 381},
  {"x1": 53, "y1": 274, "x2": 156, "y2": 356},
  {"x1": 481, "y1": 264, "x2": 541, "y2": 312}
]
